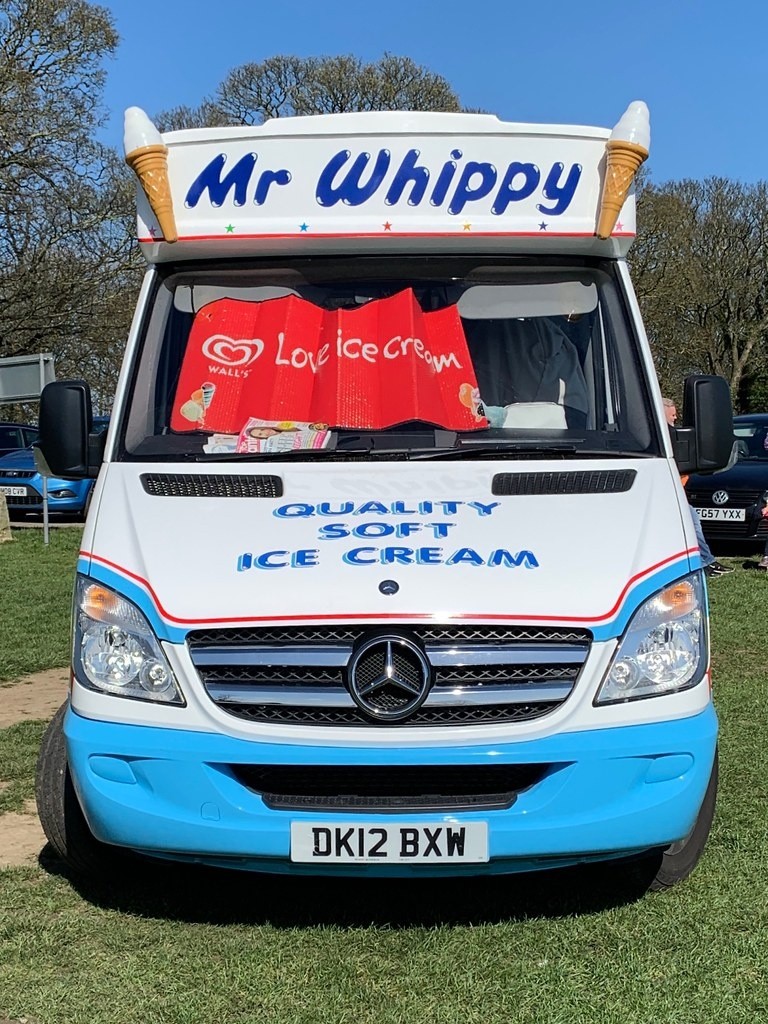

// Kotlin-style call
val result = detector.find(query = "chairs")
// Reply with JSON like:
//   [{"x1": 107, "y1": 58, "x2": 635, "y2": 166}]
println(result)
[{"x1": 470, "y1": 317, "x2": 592, "y2": 430}]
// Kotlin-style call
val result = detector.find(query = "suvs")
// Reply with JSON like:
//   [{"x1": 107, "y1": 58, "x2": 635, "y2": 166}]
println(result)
[{"x1": 0, "y1": 416, "x2": 111, "y2": 522}]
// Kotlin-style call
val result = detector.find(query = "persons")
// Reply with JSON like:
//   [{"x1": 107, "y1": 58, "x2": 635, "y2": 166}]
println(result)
[
  {"x1": 246, "y1": 421, "x2": 301, "y2": 440},
  {"x1": 662, "y1": 398, "x2": 735, "y2": 578}
]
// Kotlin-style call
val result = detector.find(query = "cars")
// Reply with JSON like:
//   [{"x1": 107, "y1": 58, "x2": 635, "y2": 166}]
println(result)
[
  {"x1": 677, "y1": 412, "x2": 768, "y2": 553},
  {"x1": 0, "y1": 424, "x2": 40, "y2": 460}
]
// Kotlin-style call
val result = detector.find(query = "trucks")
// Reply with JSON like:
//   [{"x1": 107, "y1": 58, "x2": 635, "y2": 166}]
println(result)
[{"x1": 33, "y1": 97, "x2": 734, "y2": 897}]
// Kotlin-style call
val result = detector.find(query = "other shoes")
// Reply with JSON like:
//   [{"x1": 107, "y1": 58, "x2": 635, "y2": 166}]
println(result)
[
  {"x1": 707, "y1": 572, "x2": 722, "y2": 578},
  {"x1": 709, "y1": 562, "x2": 736, "y2": 573}
]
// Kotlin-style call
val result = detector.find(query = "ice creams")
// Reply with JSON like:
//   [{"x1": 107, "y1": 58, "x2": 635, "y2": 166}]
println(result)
[
  {"x1": 596, "y1": 100, "x2": 650, "y2": 237},
  {"x1": 123, "y1": 107, "x2": 178, "y2": 244}
]
